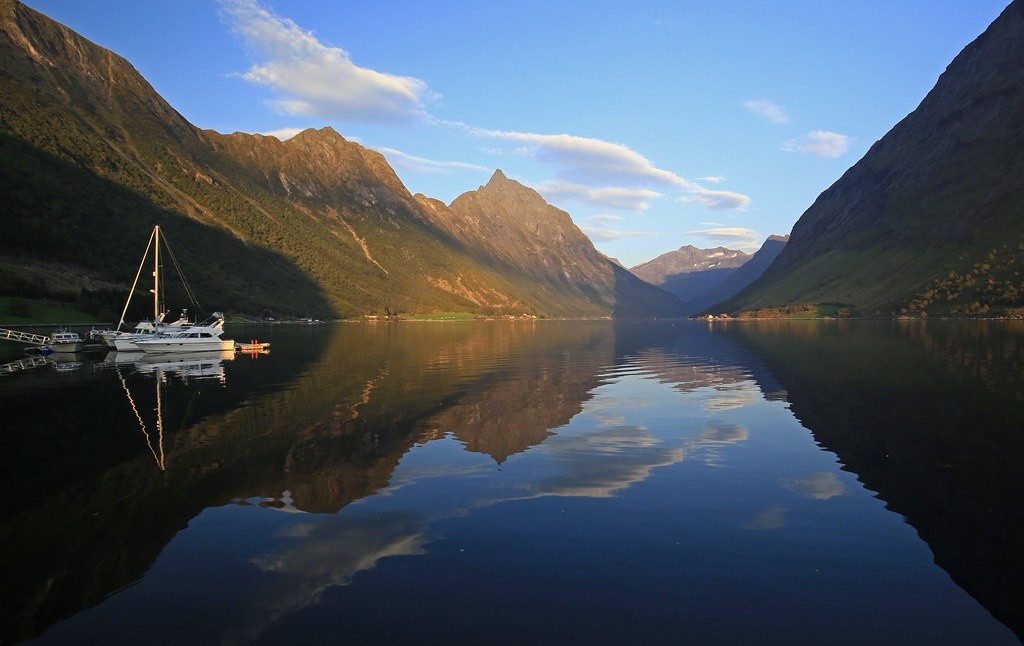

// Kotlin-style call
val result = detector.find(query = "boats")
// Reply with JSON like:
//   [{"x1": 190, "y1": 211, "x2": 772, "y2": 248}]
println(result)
[
  {"x1": 114, "y1": 352, "x2": 236, "y2": 387},
  {"x1": 45, "y1": 325, "x2": 86, "y2": 352},
  {"x1": 51, "y1": 353, "x2": 86, "y2": 372}
]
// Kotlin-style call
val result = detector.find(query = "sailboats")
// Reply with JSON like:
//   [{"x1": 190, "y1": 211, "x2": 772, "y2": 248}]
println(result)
[
  {"x1": 102, "y1": 226, "x2": 270, "y2": 353},
  {"x1": 104, "y1": 350, "x2": 270, "y2": 470}
]
